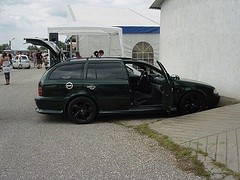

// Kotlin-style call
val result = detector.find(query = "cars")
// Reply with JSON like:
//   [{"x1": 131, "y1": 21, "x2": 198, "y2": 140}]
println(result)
[{"x1": 12, "y1": 54, "x2": 32, "y2": 69}]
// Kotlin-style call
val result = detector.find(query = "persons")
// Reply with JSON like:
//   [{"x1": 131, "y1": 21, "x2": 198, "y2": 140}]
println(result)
[
  {"x1": 94, "y1": 50, "x2": 104, "y2": 57},
  {"x1": 9, "y1": 51, "x2": 32, "y2": 64},
  {"x1": 32, "y1": 50, "x2": 48, "y2": 69},
  {"x1": 76, "y1": 52, "x2": 81, "y2": 58},
  {"x1": 65, "y1": 52, "x2": 69, "y2": 61},
  {"x1": 0, "y1": 53, "x2": 11, "y2": 85}
]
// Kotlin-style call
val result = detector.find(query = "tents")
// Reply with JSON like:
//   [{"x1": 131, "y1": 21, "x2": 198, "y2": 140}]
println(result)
[{"x1": 48, "y1": 19, "x2": 123, "y2": 67}]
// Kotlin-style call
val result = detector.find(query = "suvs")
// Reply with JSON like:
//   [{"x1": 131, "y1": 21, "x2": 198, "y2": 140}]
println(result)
[{"x1": 25, "y1": 38, "x2": 221, "y2": 125}]
